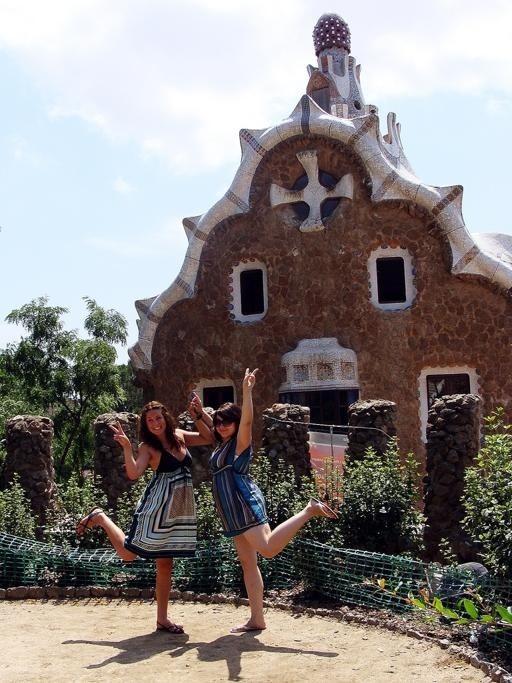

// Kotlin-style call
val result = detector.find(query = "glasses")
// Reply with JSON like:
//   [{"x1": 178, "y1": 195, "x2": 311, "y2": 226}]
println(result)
[{"x1": 213, "y1": 418, "x2": 232, "y2": 427}]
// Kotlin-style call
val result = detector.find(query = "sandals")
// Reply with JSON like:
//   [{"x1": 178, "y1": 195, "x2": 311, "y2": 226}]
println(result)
[
  {"x1": 76, "y1": 506, "x2": 104, "y2": 535},
  {"x1": 231, "y1": 619, "x2": 266, "y2": 633},
  {"x1": 306, "y1": 496, "x2": 338, "y2": 519},
  {"x1": 157, "y1": 621, "x2": 184, "y2": 634}
]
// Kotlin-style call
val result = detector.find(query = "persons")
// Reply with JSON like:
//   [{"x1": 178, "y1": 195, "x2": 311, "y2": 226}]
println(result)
[
  {"x1": 76, "y1": 400, "x2": 213, "y2": 634},
  {"x1": 192, "y1": 366, "x2": 339, "y2": 633}
]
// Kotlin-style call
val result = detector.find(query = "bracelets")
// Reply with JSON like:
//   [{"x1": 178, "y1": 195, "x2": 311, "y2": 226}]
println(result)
[
  {"x1": 191, "y1": 416, "x2": 200, "y2": 423},
  {"x1": 198, "y1": 410, "x2": 207, "y2": 418}
]
